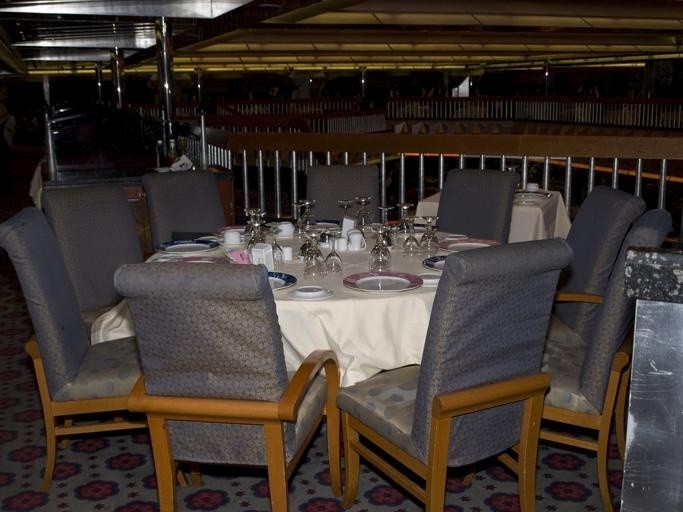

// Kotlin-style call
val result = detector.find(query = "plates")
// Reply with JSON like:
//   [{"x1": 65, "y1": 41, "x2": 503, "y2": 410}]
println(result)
[
  {"x1": 217, "y1": 226, "x2": 246, "y2": 234},
  {"x1": 421, "y1": 283, "x2": 439, "y2": 288},
  {"x1": 156, "y1": 240, "x2": 219, "y2": 253},
  {"x1": 343, "y1": 272, "x2": 423, "y2": 293},
  {"x1": 287, "y1": 286, "x2": 335, "y2": 300},
  {"x1": 423, "y1": 256, "x2": 447, "y2": 271},
  {"x1": 439, "y1": 239, "x2": 500, "y2": 252},
  {"x1": 183, "y1": 257, "x2": 228, "y2": 264},
  {"x1": 514, "y1": 190, "x2": 551, "y2": 204},
  {"x1": 192, "y1": 233, "x2": 223, "y2": 241},
  {"x1": 268, "y1": 272, "x2": 297, "y2": 291},
  {"x1": 445, "y1": 234, "x2": 468, "y2": 240}
]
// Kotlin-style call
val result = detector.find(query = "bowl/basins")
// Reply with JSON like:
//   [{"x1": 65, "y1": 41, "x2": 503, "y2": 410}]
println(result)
[{"x1": 419, "y1": 273, "x2": 440, "y2": 284}]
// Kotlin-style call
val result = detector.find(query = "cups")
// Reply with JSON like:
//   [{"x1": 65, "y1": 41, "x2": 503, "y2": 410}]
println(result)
[
  {"x1": 347, "y1": 229, "x2": 360, "y2": 240},
  {"x1": 224, "y1": 232, "x2": 240, "y2": 243},
  {"x1": 350, "y1": 233, "x2": 366, "y2": 252},
  {"x1": 252, "y1": 244, "x2": 273, "y2": 270},
  {"x1": 527, "y1": 183, "x2": 538, "y2": 190}
]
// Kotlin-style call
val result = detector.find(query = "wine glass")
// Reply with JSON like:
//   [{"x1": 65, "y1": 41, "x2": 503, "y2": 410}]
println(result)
[
  {"x1": 353, "y1": 192, "x2": 374, "y2": 227},
  {"x1": 378, "y1": 206, "x2": 394, "y2": 233},
  {"x1": 338, "y1": 199, "x2": 353, "y2": 226},
  {"x1": 244, "y1": 208, "x2": 261, "y2": 233},
  {"x1": 299, "y1": 200, "x2": 315, "y2": 228},
  {"x1": 247, "y1": 210, "x2": 265, "y2": 248},
  {"x1": 301, "y1": 230, "x2": 311, "y2": 255},
  {"x1": 302, "y1": 229, "x2": 326, "y2": 283},
  {"x1": 292, "y1": 203, "x2": 305, "y2": 237},
  {"x1": 325, "y1": 229, "x2": 343, "y2": 272},
  {"x1": 364, "y1": 226, "x2": 392, "y2": 272},
  {"x1": 419, "y1": 216, "x2": 439, "y2": 252},
  {"x1": 265, "y1": 222, "x2": 284, "y2": 269},
  {"x1": 397, "y1": 204, "x2": 413, "y2": 234},
  {"x1": 306, "y1": 241, "x2": 321, "y2": 256},
  {"x1": 401, "y1": 218, "x2": 420, "y2": 250}
]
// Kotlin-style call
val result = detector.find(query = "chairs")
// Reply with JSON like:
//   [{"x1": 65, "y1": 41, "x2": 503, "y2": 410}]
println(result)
[
  {"x1": 303, "y1": 163, "x2": 380, "y2": 227},
  {"x1": 41, "y1": 186, "x2": 143, "y2": 336},
  {"x1": 436, "y1": 168, "x2": 520, "y2": 243},
  {"x1": 0, "y1": 208, "x2": 189, "y2": 492},
  {"x1": 142, "y1": 171, "x2": 226, "y2": 249},
  {"x1": 335, "y1": 237, "x2": 573, "y2": 512},
  {"x1": 544, "y1": 185, "x2": 646, "y2": 351},
  {"x1": 512, "y1": 209, "x2": 673, "y2": 512},
  {"x1": 115, "y1": 263, "x2": 343, "y2": 511}
]
